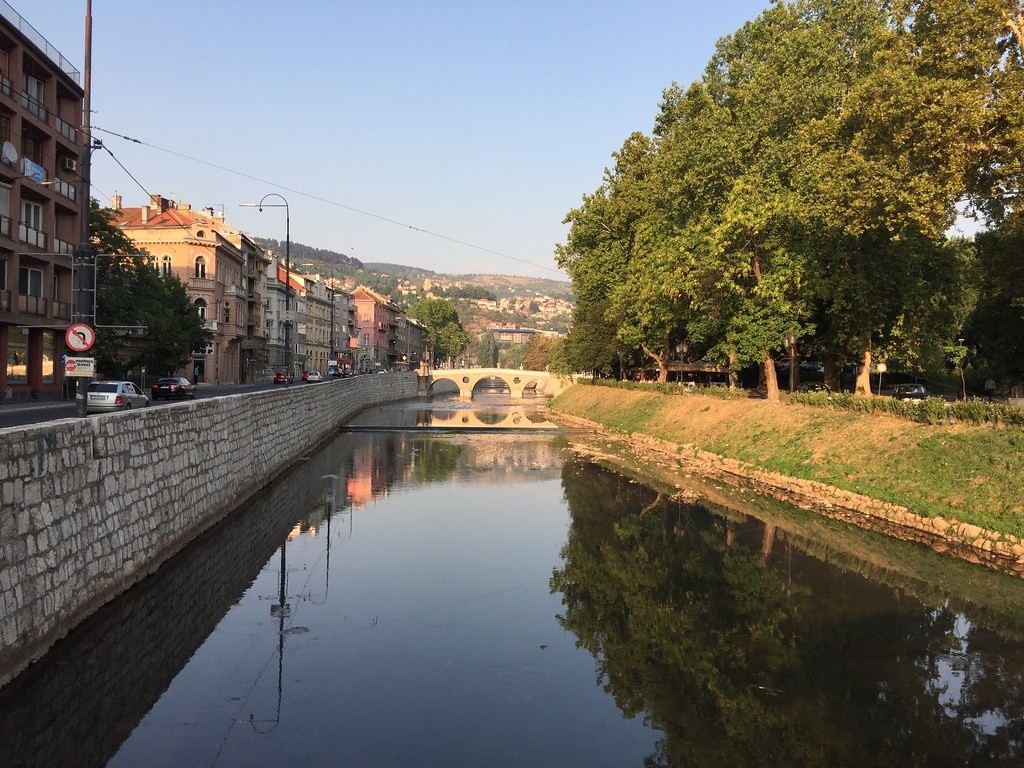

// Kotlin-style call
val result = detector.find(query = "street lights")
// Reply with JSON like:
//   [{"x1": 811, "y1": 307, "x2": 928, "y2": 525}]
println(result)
[
  {"x1": 308, "y1": 503, "x2": 331, "y2": 605},
  {"x1": 250, "y1": 540, "x2": 287, "y2": 736},
  {"x1": 239, "y1": 193, "x2": 295, "y2": 386},
  {"x1": 303, "y1": 264, "x2": 336, "y2": 366},
  {"x1": 338, "y1": 495, "x2": 353, "y2": 539}
]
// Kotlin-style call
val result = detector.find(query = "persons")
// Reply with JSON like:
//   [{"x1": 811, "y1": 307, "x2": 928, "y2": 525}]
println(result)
[
  {"x1": 633, "y1": 373, "x2": 658, "y2": 381},
  {"x1": 193, "y1": 365, "x2": 199, "y2": 385},
  {"x1": 284, "y1": 365, "x2": 295, "y2": 384},
  {"x1": 622, "y1": 373, "x2": 627, "y2": 381}
]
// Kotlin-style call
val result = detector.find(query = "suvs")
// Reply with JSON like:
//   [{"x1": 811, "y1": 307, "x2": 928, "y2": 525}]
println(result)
[{"x1": 307, "y1": 371, "x2": 324, "y2": 383}]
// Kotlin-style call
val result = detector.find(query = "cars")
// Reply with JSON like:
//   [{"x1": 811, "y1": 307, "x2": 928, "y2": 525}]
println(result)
[
  {"x1": 892, "y1": 384, "x2": 931, "y2": 401},
  {"x1": 87, "y1": 381, "x2": 149, "y2": 413},
  {"x1": 794, "y1": 382, "x2": 832, "y2": 395},
  {"x1": 342, "y1": 370, "x2": 352, "y2": 378},
  {"x1": 370, "y1": 369, "x2": 377, "y2": 374},
  {"x1": 302, "y1": 371, "x2": 310, "y2": 381},
  {"x1": 274, "y1": 372, "x2": 293, "y2": 384},
  {"x1": 151, "y1": 377, "x2": 196, "y2": 401},
  {"x1": 377, "y1": 370, "x2": 385, "y2": 374}
]
